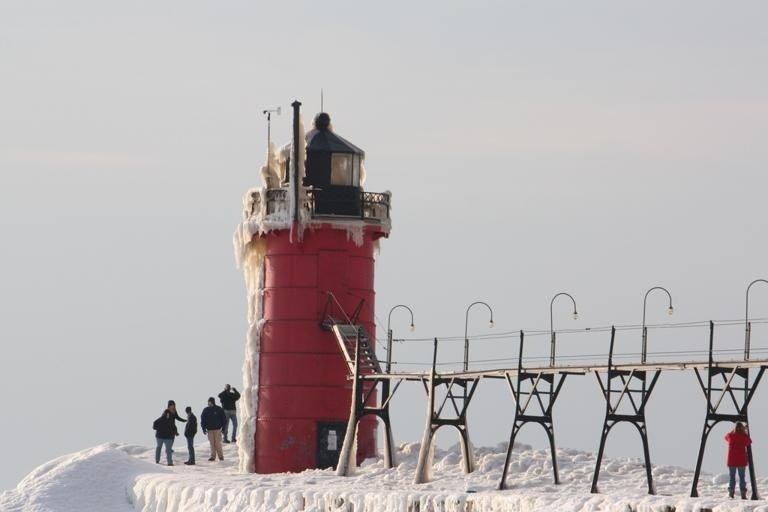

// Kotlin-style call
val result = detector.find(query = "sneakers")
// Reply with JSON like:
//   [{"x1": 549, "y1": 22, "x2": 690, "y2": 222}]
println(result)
[{"x1": 156, "y1": 435, "x2": 237, "y2": 465}]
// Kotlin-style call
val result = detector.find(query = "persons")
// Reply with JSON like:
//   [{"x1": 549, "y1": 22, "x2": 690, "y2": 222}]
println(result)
[
  {"x1": 184, "y1": 406, "x2": 197, "y2": 465},
  {"x1": 167, "y1": 400, "x2": 187, "y2": 450},
  {"x1": 724, "y1": 422, "x2": 752, "y2": 500},
  {"x1": 218, "y1": 384, "x2": 241, "y2": 443},
  {"x1": 154, "y1": 409, "x2": 179, "y2": 466},
  {"x1": 200, "y1": 397, "x2": 226, "y2": 461}
]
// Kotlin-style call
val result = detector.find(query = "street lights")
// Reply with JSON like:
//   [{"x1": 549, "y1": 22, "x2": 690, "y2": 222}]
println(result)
[
  {"x1": 642, "y1": 286, "x2": 674, "y2": 365},
  {"x1": 463, "y1": 301, "x2": 493, "y2": 372},
  {"x1": 385, "y1": 306, "x2": 414, "y2": 370},
  {"x1": 550, "y1": 293, "x2": 578, "y2": 367}
]
